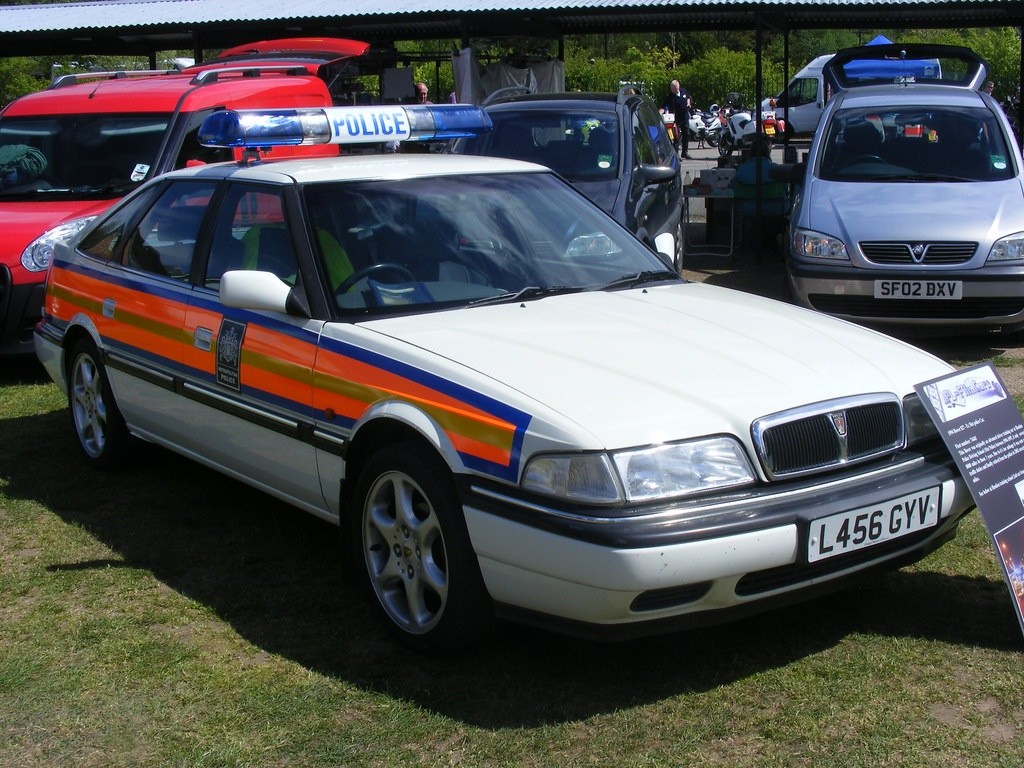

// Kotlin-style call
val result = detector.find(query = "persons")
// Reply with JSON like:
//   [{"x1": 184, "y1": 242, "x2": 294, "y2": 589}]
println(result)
[
  {"x1": 416, "y1": 82, "x2": 433, "y2": 105},
  {"x1": 1012, "y1": 87, "x2": 1020, "y2": 130},
  {"x1": 658, "y1": 80, "x2": 692, "y2": 162},
  {"x1": 981, "y1": 81, "x2": 1000, "y2": 104}
]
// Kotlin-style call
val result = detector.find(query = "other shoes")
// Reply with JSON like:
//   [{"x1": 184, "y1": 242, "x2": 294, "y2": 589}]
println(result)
[
  {"x1": 678, "y1": 156, "x2": 683, "y2": 162},
  {"x1": 681, "y1": 153, "x2": 692, "y2": 159}
]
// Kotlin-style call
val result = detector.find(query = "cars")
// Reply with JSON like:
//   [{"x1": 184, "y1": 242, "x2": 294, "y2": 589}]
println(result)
[{"x1": 786, "y1": 43, "x2": 1024, "y2": 348}]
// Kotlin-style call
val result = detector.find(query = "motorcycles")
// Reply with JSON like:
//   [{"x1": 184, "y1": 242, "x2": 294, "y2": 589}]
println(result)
[{"x1": 665, "y1": 91, "x2": 788, "y2": 160}]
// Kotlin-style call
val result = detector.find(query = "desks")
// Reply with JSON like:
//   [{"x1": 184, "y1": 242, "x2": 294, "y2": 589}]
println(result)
[{"x1": 683, "y1": 181, "x2": 748, "y2": 257}]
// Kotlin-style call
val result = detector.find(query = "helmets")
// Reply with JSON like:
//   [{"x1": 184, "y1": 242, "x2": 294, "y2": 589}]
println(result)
[{"x1": 710, "y1": 104, "x2": 721, "y2": 114}]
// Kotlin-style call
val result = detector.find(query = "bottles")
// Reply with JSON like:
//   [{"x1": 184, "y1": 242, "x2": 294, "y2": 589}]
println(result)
[{"x1": 665, "y1": 107, "x2": 669, "y2": 114}]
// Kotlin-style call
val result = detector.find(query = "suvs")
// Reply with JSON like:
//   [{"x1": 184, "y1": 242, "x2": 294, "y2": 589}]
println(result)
[
  {"x1": 0, "y1": 35, "x2": 371, "y2": 380},
  {"x1": 441, "y1": 85, "x2": 683, "y2": 278}
]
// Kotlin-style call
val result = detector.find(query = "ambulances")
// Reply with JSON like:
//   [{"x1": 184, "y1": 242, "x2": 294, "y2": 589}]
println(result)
[{"x1": 33, "y1": 104, "x2": 980, "y2": 651}]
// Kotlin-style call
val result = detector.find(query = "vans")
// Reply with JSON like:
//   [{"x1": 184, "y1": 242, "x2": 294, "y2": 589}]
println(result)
[{"x1": 754, "y1": 54, "x2": 942, "y2": 143}]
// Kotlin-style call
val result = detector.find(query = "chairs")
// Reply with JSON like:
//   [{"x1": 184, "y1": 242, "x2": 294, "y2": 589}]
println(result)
[
  {"x1": 493, "y1": 128, "x2": 614, "y2": 175},
  {"x1": 153, "y1": 196, "x2": 535, "y2": 317},
  {"x1": 830, "y1": 112, "x2": 996, "y2": 181},
  {"x1": 736, "y1": 180, "x2": 787, "y2": 248}
]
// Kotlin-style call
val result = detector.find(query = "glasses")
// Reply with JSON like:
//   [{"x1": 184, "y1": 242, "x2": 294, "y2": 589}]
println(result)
[{"x1": 987, "y1": 86, "x2": 993, "y2": 89}]
